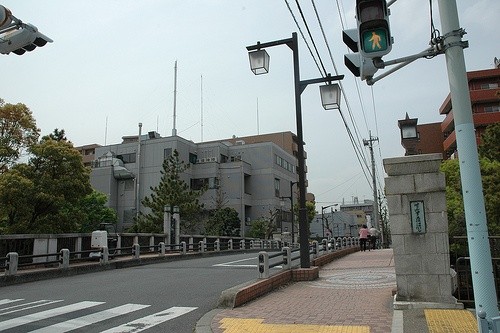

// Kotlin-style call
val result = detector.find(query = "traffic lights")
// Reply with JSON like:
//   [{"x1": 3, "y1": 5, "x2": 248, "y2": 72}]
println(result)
[
  {"x1": 354, "y1": 0, "x2": 394, "y2": 59},
  {"x1": 342, "y1": 27, "x2": 378, "y2": 82}
]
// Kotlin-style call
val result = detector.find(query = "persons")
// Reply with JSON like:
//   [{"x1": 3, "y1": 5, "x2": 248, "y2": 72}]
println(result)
[
  {"x1": 358, "y1": 224, "x2": 368, "y2": 252},
  {"x1": 369, "y1": 225, "x2": 380, "y2": 250}
]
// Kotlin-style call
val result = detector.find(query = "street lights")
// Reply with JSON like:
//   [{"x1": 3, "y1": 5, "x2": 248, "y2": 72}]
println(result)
[
  {"x1": 321, "y1": 203, "x2": 338, "y2": 240},
  {"x1": 289, "y1": 180, "x2": 309, "y2": 248},
  {"x1": 246, "y1": 31, "x2": 345, "y2": 269}
]
